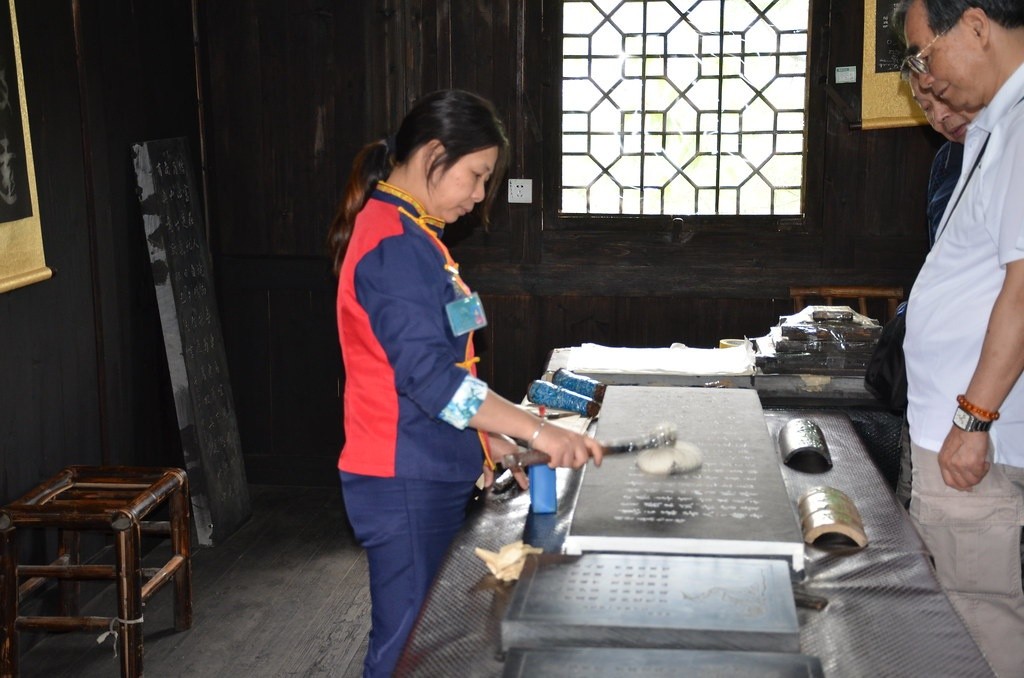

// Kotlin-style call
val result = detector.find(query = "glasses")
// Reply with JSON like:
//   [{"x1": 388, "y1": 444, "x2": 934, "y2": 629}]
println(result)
[{"x1": 907, "y1": 24, "x2": 951, "y2": 74}]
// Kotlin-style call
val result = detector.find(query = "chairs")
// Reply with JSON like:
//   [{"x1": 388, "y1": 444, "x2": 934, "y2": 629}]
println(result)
[{"x1": 791, "y1": 288, "x2": 905, "y2": 327}]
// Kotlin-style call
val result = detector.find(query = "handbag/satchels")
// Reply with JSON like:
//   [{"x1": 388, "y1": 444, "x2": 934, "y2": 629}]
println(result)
[{"x1": 863, "y1": 301, "x2": 909, "y2": 417}]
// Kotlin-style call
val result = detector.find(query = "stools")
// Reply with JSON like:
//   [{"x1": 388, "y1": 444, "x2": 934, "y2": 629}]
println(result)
[{"x1": 0, "y1": 464, "x2": 193, "y2": 678}]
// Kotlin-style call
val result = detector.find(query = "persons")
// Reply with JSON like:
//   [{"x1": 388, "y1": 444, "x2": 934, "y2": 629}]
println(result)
[
  {"x1": 335, "y1": 90, "x2": 602, "y2": 678},
  {"x1": 896, "y1": 55, "x2": 970, "y2": 510},
  {"x1": 890, "y1": 0, "x2": 1024, "y2": 678},
  {"x1": 474, "y1": 309, "x2": 484, "y2": 324}
]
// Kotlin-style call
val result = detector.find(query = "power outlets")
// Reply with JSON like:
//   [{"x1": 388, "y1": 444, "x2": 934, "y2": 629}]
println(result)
[{"x1": 508, "y1": 178, "x2": 533, "y2": 204}]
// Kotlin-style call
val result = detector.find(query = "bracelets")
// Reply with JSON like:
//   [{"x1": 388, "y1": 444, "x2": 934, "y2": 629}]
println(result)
[
  {"x1": 956, "y1": 394, "x2": 1000, "y2": 421},
  {"x1": 529, "y1": 417, "x2": 547, "y2": 449}
]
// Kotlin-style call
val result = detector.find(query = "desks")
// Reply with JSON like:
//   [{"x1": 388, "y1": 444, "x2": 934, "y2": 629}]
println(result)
[{"x1": 393, "y1": 344, "x2": 999, "y2": 678}]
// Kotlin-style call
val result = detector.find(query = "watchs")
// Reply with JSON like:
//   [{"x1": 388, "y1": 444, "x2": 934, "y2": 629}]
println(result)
[{"x1": 952, "y1": 407, "x2": 992, "y2": 432}]
